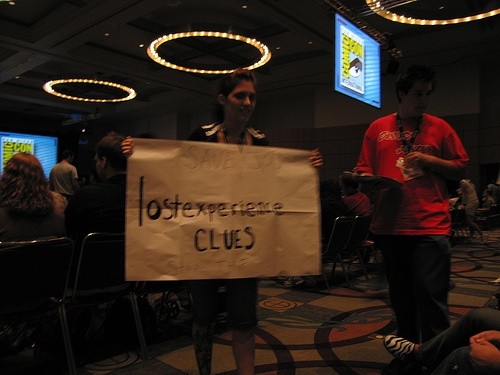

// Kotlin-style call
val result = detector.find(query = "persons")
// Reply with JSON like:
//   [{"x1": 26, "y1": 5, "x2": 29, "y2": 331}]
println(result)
[
  {"x1": 120, "y1": 68, "x2": 324, "y2": 375},
  {"x1": 0, "y1": 151, "x2": 68, "y2": 241},
  {"x1": 343, "y1": 64, "x2": 470, "y2": 344},
  {"x1": 384, "y1": 307, "x2": 500, "y2": 367},
  {"x1": 479, "y1": 183, "x2": 500, "y2": 218},
  {"x1": 48, "y1": 149, "x2": 80, "y2": 200},
  {"x1": 435, "y1": 330, "x2": 500, "y2": 375},
  {"x1": 339, "y1": 172, "x2": 371, "y2": 217},
  {"x1": 460, "y1": 179, "x2": 487, "y2": 243},
  {"x1": 281, "y1": 171, "x2": 345, "y2": 288},
  {"x1": 65, "y1": 133, "x2": 127, "y2": 343}
]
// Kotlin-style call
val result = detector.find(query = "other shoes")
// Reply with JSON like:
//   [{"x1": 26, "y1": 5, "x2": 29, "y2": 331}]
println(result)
[
  {"x1": 463, "y1": 240, "x2": 472, "y2": 244},
  {"x1": 480, "y1": 238, "x2": 485, "y2": 244}
]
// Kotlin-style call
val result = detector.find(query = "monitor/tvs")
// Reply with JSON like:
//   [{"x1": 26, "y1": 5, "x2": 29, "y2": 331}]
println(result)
[{"x1": 333, "y1": 13, "x2": 384, "y2": 110}]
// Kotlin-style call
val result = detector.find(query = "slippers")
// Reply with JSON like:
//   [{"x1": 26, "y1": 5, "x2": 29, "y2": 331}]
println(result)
[{"x1": 384, "y1": 335, "x2": 423, "y2": 368}]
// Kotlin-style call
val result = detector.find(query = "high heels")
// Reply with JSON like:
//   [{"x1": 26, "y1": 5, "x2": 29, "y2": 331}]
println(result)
[{"x1": 31, "y1": 341, "x2": 48, "y2": 358}]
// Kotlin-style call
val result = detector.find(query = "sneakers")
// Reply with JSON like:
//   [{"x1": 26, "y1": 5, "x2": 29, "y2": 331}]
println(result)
[{"x1": 283, "y1": 276, "x2": 304, "y2": 287}]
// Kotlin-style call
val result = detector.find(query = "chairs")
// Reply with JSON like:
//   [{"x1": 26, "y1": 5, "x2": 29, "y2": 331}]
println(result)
[
  {"x1": 321, "y1": 215, "x2": 374, "y2": 296},
  {"x1": 0, "y1": 236, "x2": 78, "y2": 375},
  {"x1": 449, "y1": 204, "x2": 479, "y2": 243},
  {"x1": 72, "y1": 231, "x2": 149, "y2": 361}
]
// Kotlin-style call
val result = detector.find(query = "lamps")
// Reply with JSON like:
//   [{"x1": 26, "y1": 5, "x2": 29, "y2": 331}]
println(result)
[
  {"x1": 41, "y1": 73, "x2": 139, "y2": 104},
  {"x1": 146, "y1": 21, "x2": 273, "y2": 75},
  {"x1": 365, "y1": 0, "x2": 500, "y2": 26}
]
